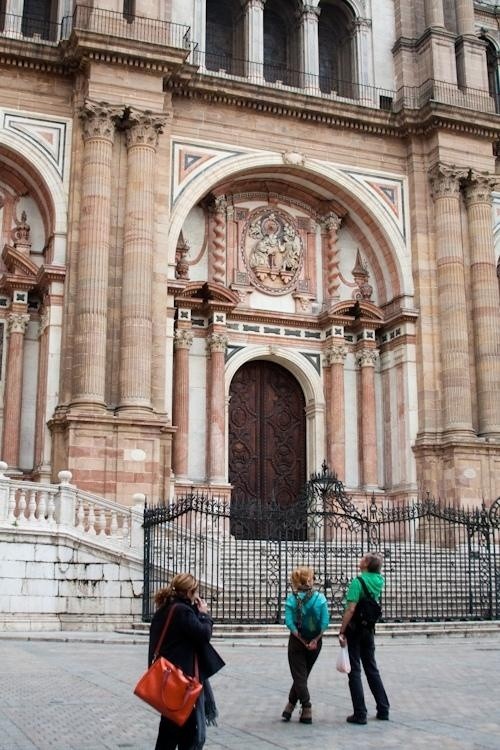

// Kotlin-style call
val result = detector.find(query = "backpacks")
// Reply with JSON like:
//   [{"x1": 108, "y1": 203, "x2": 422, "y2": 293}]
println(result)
[{"x1": 294, "y1": 591, "x2": 321, "y2": 640}]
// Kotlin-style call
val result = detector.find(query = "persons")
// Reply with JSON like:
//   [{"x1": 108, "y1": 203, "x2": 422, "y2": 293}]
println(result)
[
  {"x1": 248, "y1": 237, "x2": 272, "y2": 267},
  {"x1": 279, "y1": 566, "x2": 331, "y2": 724},
  {"x1": 249, "y1": 220, "x2": 264, "y2": 235},
  {"x1": 147, "y1": 570, "x2": 226, "y2": 749},
  {"x1": 275, "y1": 233, "x2": 297, "y2": 272},
  {"x1": 335, "y1": 551, "x2": 393, "y2": 725}
]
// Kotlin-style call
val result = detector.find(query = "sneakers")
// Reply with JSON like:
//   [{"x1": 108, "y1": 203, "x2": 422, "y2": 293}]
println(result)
[
  {"x1": 346, "y1": 715, "x2": 368, "y2": 725},
  {"x1": 376, "y1": 711, "x2": 389, "y2": 719}
]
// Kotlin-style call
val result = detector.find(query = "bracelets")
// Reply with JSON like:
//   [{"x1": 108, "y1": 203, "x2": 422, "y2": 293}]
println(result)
[{"x1": 340, "y1": 631, "x2": 344, "y2": 635}]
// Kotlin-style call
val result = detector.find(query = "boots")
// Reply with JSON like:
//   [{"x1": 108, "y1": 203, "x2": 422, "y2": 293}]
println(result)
[
  {"x1": 283, "y1": 703, "x2": 295, "y2": 720},
  {"x1": 299, "y1": 708, "x2": 312, "y2": 724}
]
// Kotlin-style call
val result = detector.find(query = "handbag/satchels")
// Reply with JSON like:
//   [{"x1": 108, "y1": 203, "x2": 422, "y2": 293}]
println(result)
[
  {"x1": 351, "y1": 595, "x2": 381, "y2": 632},
  {"x1": 133, "y1": 656, "x2": 204, "y2": 727}
]
